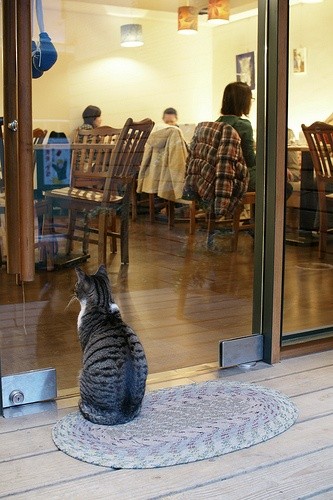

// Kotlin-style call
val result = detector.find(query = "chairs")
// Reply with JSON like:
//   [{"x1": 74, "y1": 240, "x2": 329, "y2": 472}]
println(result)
[{"x1": 0, "y1": 118, "x2": 333, "y2": 273}]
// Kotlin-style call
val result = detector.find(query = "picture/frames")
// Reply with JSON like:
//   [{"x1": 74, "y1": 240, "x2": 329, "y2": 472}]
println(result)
[
  {"x1": 291, "y1": 48, "x2": 306, "y2": 73},
  {"x1": 234, "y1": 52, "x2": 255, "y2": 90}
]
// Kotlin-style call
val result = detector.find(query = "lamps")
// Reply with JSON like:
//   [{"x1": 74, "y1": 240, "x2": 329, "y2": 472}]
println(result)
[
  {"x1": 178, "y1": 0, "x2": 198, "y2": 34},
  {"x1": 207, "y1": 0, "x2": 231, "y2": 22},
  {"x1": 120, "y1": 0, "x2": 145, "y2": 48}
]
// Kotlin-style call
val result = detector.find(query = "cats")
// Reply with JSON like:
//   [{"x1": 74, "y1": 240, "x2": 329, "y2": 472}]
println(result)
[{"x1": 62, "y1": 262, "x2": 149, "y2": 427}]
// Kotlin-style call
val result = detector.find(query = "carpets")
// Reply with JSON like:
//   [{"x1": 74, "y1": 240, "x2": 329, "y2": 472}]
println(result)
[{"x1": 52, "y1": 383, "x2": 295, "y2": 470}]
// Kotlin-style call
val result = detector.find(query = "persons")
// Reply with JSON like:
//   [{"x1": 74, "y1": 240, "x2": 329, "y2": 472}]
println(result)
[
  {"x1": 217, "y1": 81, "x2": 293, "y2": 239},
  {"x1": 78, "y1": 105, "x2": 103, "y2": 167},
  {"x1": 162, "y1": 105, "x2": 177, "y2": 127}
]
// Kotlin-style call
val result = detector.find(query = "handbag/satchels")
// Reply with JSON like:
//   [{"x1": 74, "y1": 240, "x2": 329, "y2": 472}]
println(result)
[
  {"x1": 181, "y1": 185, "x2": 195, "y2": 200},
  {"x1": 30, "y1": 33, "x2": 57, "y2": 79}
]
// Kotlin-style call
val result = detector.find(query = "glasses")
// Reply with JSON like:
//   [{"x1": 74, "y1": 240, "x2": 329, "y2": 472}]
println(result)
[{"x1": 250, "y1": 98, "x2": 256, "y2": 102}]
